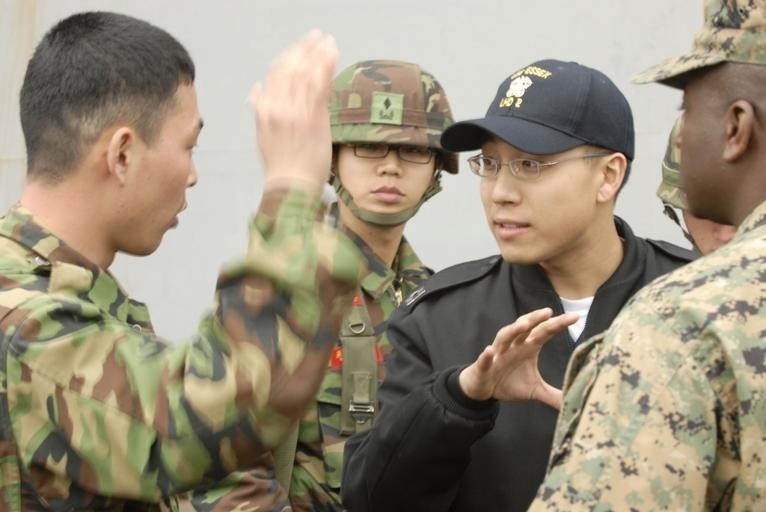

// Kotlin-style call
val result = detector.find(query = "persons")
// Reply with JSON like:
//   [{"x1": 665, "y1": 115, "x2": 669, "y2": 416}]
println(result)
[
  {"x1": 656, "y1": 115, "x2": 737, "y2": 256},
  {"x1": 0, "y1": 11, "x2": 369, "y2": 512},
  {"x1": 341, "y1": 60, "x2": 703, "y2": 512},
  {"x1": 188, "y1": 60, "x2": 460, "y2": 512},
  {"x1": 527, "y1": 0, "x2": 766, "y2": 512}
]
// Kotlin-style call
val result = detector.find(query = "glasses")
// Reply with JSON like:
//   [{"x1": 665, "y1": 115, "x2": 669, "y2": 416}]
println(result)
[
  {"x1": 346, "y1": 142, "x2": 435, "y2": 166},
  {"x1": 466, "y1": 152, "x2": 599, "y2": 184}
]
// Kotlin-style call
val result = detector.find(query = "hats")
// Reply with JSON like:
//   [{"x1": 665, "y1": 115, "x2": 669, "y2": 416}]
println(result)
[
  {"x1": 440, "y1": 59, "x2": 636, "y2": 164},
  {"x1": 630, "y1": 0, "x2": 763, "y2": 87}
]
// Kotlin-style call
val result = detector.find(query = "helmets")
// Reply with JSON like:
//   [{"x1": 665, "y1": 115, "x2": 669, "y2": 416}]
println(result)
[{"x1": 325, "y1": 59, "x2": 460, "y2": 175}]
